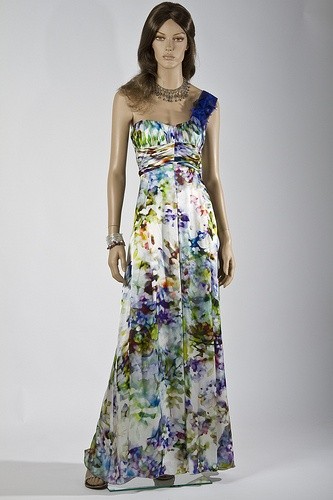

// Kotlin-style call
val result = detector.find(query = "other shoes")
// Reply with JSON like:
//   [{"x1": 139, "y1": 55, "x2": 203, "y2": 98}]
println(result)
[
  {"x1": 153, "y1": 475, "x2": 175, "y2": 486},
  {"x1": 84, "y1": 469, "x2": 109, "y2": 490}
]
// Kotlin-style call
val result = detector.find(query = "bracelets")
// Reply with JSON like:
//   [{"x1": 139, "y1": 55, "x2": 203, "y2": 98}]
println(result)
[{"x1": 106, "y1": 231, "x2": 125, "y2": 251}]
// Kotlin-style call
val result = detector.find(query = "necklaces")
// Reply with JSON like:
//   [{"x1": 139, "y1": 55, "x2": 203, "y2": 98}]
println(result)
[{"x1": 149, "y1": 74, "x2": 190, "y2": 103}]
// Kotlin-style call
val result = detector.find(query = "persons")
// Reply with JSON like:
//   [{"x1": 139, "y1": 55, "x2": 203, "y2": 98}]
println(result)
[{"x1": 83, "y1": 2, "x2": 239, "y2": 489}]
